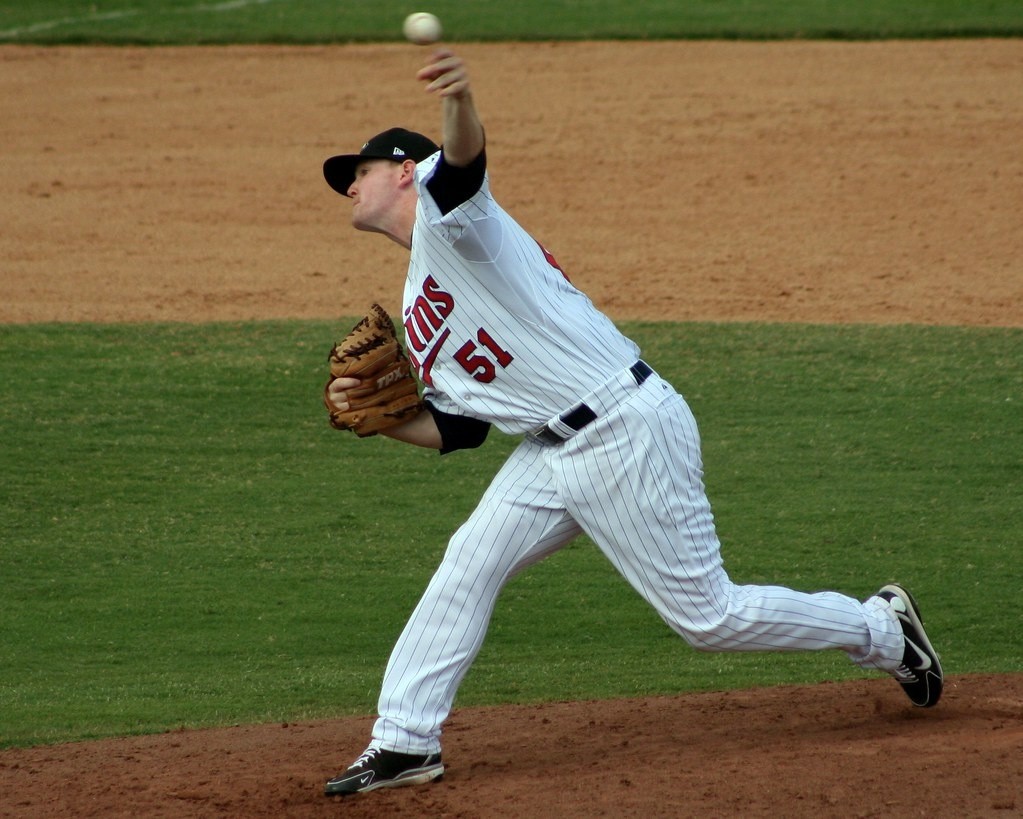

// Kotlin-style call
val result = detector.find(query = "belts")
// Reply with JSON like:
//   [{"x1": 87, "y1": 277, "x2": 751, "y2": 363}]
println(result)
[{"x1": 525, "y1": 361, "x2": 653, "y2": 446}]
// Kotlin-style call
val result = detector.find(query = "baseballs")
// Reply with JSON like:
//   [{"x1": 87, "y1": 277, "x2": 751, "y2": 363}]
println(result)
[{"x1": 401, "y1": 11, "x2": 442, "y2": 46}]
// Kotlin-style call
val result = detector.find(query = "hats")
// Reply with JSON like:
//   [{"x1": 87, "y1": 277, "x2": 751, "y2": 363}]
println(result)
[{"x1": 323, "y1": 128, "x2": 441, "y2": 198}]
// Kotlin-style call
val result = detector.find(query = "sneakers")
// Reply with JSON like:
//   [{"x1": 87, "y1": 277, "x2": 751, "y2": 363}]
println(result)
[
  {"x1": 324, "y1": 745, "x2": 444, "y2": 799},
  {"x1": 873, "y1": 583, "x2": 944, "y2": 709}
]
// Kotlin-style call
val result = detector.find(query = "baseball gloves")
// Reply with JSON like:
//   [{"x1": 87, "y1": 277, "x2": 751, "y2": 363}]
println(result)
[{"x1": 322, "y1": 301, "x2": 423, "y2": 437}]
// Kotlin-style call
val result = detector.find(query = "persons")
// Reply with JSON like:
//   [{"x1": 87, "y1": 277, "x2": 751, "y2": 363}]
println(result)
[{"x1": 322, "y1": 48, "x2": 943, "y2": 797}]
